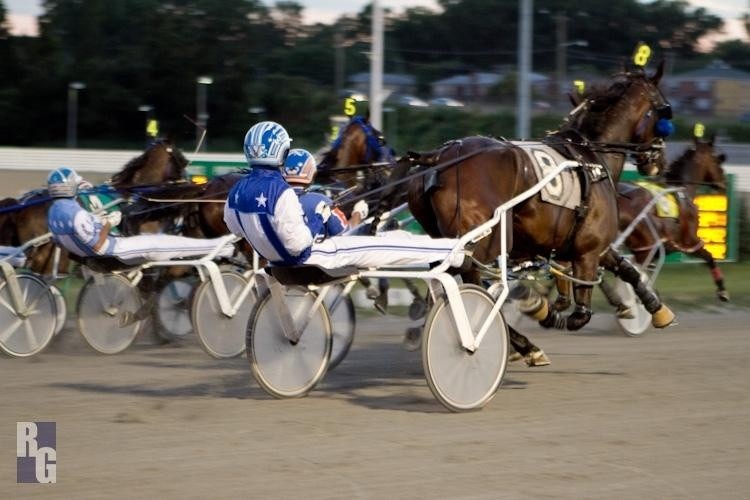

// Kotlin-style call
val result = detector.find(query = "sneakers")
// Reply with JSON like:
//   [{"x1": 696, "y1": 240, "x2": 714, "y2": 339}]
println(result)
[
  {"x1": 458, "y1": 238, "x2": 481, "y2": 273},
  {"x1": 232, "y1": 248, "x2": 239, "y2": 257}
]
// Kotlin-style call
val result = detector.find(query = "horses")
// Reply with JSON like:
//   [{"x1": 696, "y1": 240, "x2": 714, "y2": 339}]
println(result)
[
  {"x1": 120, "y1": 108, "x2": 398, "y2": 346},
  {"x1": 378, "y1": 63, "x2": 731, "y2": 367},
  {"x1": 0, "y1": 133, "x2": 187, "y2": 351}
]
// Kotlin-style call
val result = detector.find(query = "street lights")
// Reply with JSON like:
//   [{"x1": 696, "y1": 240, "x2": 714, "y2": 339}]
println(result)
[
  {"x1": 553, "y1": 39, "x2": 589, "y2": 104},
  {"x1": 63, "y1": 79, "x2": 85, "y2": 145},
  {"x1": 196, "y1": 74, "x2": 215, "y2": 153}
]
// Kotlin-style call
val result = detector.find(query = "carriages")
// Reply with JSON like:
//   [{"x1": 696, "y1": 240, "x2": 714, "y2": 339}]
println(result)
[
  {"x1": 2, "y1": 106, "x2": 729, "y2": 413},
  {"x1": 236, "y1": 46, "x2": 675, "y2": 410}
]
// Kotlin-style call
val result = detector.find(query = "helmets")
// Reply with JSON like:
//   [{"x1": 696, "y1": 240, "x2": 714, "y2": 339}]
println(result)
[
  {"x1": 46, "y1": 166, "x2": 84, "y2": 198},
  {"x1": 280, "y1": 148, "x2": 318, "y2": 185},
  {"x1": 242, "y1": 120, "x2": 293, "y2": 167}
]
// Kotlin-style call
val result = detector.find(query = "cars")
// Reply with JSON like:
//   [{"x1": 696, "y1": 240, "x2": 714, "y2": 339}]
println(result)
[{"x1": 335, "y1": 83, "x2": 472, "y2": 114}]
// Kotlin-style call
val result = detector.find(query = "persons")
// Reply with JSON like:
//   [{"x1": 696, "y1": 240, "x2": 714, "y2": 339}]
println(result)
[
  {"x1": 48, "y1": 166, "x2": 241, "y2": 265},
  {"x1": 1, "y1": 247, "x2": 34, "y2": 267},
  {"x1": 282, "y1": 148, "x2": 398, "y2": 240},
  {"x1": 224, "y1": 120, "x2": 479, "y2": 279}
]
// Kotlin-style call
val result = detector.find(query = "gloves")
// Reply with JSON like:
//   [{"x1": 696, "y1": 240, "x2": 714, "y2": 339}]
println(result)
[
  {"x1": 106, "y1": 210, "x2": 123, "y2": 228},
  {"x1": 351, "y1": 199, "x2": 372, "y2": 219},
  {"x1": 315, "y1": 199, "x2": 332, "y2": 224}
]
